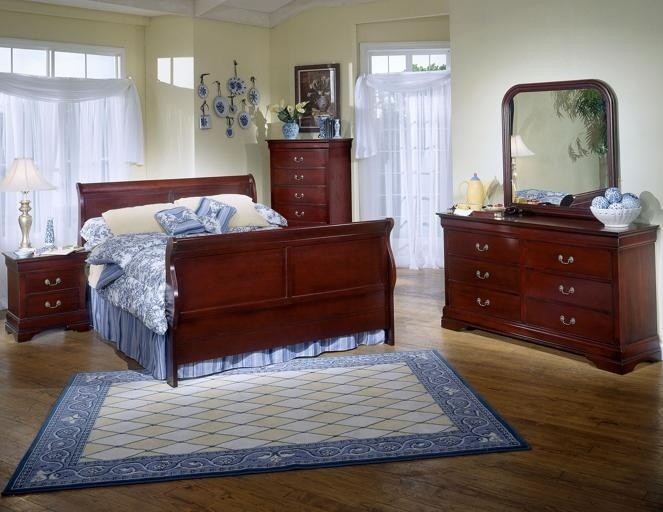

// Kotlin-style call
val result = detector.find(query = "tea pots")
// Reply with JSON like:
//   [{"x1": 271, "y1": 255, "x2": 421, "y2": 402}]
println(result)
[{"x1": 458, "y1": 172, "x2": 490, "y2": 212}]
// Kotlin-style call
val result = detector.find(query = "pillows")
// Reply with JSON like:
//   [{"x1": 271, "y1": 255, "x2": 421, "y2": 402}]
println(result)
[{"x1": 101, "y1": 192, "x2": 271, "y2": 238}]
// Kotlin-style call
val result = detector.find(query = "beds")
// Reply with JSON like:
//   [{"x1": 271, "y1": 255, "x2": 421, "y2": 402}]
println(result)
[{"x1": 74, "y1": 172, "x2": 398, "y2": 390}]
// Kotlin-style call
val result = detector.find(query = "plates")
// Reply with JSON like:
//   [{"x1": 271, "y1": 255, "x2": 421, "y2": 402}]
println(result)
[
  {"x1": 200, "y1": 114, "x2": 212, "y2": 129},
  {"x1": 227, "y1": 77, "x2": 247, "y2": 95},
  {"x1": 230, "y1": 104, "x2": 238, "y2": 112},
  {"x1": 212, "y1": 96, "x2": 230, "y2": 118},
  {"x1": 249, "y1": 87, "x2": 261, "y2": 106},
  {"x1": 225, "y1": 128, "x2": 233, "y2": 138},
  {"x1": 239, "y1": 112, "x2": 250, "y2": 128},
  {"x1": 198, "y1": 84, "x2": 209, "y2": 99}
]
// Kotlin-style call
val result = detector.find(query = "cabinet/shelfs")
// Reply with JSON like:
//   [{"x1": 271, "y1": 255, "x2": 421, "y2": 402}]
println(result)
[
  {"x1": 264, "y1": 138, "x2": 354, "y2": 229},
  {"x1": 434, "y1": 211, "x2": 663, "y2": 375}
]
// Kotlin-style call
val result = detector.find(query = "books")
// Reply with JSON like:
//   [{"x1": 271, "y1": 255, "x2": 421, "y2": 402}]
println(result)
[
  {"x1": 39, "y1": 248, "x2": 76, "y2": 256},
  {"x1": 63, "y1": 246, "x2": 85, "y2": 251}
]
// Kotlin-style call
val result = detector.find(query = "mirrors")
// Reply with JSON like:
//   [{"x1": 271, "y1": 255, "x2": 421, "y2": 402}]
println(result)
[{"x1": 501, "y1": 78, "x2": 620, "y2": 220}]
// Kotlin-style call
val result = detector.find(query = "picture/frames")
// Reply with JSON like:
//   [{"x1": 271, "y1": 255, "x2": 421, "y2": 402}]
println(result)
[{"x1": 293, "y1": 62, "x2": 341, "y2": 132}]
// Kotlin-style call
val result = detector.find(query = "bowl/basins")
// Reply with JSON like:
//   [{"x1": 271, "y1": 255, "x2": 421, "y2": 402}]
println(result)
[
  {"x1": 589, "y1": 204, "x2": 642, "y2": 231},
  {"x1": 14, "y1": 247, "x2": 35, "y2": 258}
]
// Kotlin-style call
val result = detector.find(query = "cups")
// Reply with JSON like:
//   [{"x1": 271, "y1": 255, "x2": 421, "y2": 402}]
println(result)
[{"x1": 453, "y1": 203, "x2": 483, "y2": 212}]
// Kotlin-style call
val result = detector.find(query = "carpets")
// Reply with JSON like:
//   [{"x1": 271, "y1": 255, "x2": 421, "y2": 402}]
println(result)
[{"x1": 0, "y1": 349, "x2": 533, "y2": 499}]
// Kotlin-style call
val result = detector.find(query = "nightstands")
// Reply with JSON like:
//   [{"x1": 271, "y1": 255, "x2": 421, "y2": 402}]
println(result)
[{"x1": 0, "y1": 250, "x2": 93, "y2": 343}]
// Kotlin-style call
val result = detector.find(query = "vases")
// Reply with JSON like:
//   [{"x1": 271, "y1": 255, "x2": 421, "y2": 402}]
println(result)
[{"x1": 282, "y1": 123, "x2": 300, "y2": 140}]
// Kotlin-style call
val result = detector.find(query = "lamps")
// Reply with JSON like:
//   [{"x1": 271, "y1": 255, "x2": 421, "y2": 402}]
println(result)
[
  {"x1": 511, "y1": 135, "x2": 535, "y2": 193},
  {"x1": 0, "y1": 158, "x2": 57, "y2": 254}
]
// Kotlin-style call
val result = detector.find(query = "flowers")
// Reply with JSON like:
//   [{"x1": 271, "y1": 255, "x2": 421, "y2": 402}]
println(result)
[{"x1": 267, "y1": 96, "x2": 310, "y2": 122}]
